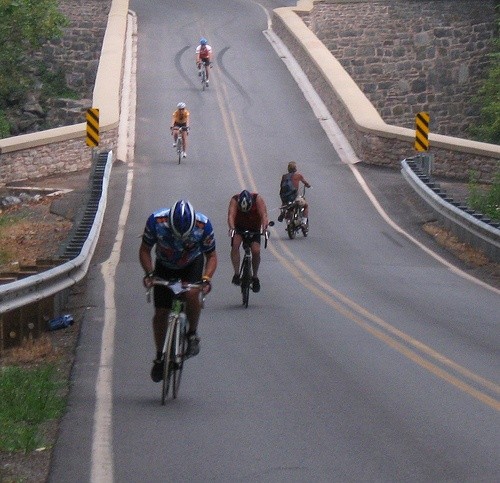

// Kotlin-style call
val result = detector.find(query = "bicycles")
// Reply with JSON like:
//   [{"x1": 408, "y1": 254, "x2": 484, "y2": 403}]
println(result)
[
  {"x1": 196, "y1": 61, "x2": 213, "y2": 92},
  {"x1": 278, "y1": 184, "x2": 311, "y2": 240},
  {"x1": 170, "y1": 126, "x2": 189, "y2": 165},
  {"x1": 230, "y1": 221, "x2": 275, "y2": 309},
  {"x1": 144, "y1": 273, "x2": 212, "y2": 406}
]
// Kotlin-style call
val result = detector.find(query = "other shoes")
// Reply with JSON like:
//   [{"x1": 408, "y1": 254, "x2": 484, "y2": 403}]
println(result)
[
  {"x1": 252, "y1": 279, "x2": 260, "y2": 292},
  {"x1": 199, "y1": 71, "x2": 201, "y2": 76},
  {"x1": 206, "y1": 82, "x2": 208, "y2": 87},
  {"x1": 278, "y1": 214, "x2": 283, "y2": 222},
  {"x1": 151, "y1": 363, "x2": 161, "y2": 382},
  {"x1": 232, "y1": 275, "x2": 241, "y2": 284},
  {"x1": 301, "y1": 222, "x2": 307, "y2": 229},
  {"x1": 173, "y1": 143, "x2": 177, "y2": 146},
  {"x1": 188, "y1": 336, "x2": 200, "y2": 356},
  {"x1": 182, "y1": 152, "x2": 186, "y2": 158}
]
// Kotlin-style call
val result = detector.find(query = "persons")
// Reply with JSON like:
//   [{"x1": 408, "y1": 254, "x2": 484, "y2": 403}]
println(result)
[
  {"x1": 170, "y1": 102, "x2": 189, "y2": 157},
  {"x1": 196, "y1": 39, "x2": 212, "y2": 84},
  {"x1": 227, "y1": 190, "x2": 268, "y2": 293},
  {"x1": 278, "y1": 162, "x2": 310, "y2": 230},
  {"x1": 138, "y1": 200, "x2": 218, "y2": 382}
]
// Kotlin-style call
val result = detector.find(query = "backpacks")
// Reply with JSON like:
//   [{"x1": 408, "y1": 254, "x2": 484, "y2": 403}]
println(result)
[{"x1": 280, "y1": 179, "x2": 296, "y2": 201}]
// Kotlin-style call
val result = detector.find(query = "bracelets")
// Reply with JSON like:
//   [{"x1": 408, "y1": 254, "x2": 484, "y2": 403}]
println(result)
[{"x1": 203, "y1": 276, "x2": 209, "y2": 280}]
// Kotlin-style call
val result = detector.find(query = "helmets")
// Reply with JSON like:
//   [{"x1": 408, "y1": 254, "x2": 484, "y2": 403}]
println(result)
[
  {"x1": 177, "y1": 102, "x2": 185, "y2": 109},
  {"x1": 170, "y1": 200, "x2": 195, "y2": 237},
  {"x1": 199, "y1": 39, "x2": 207, "y2": 44},
  {"x1": 239, "y1": 190, "x2": 252, "y2": 213}
]
